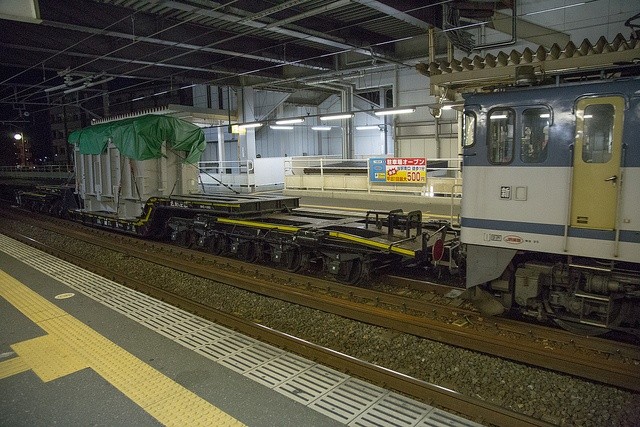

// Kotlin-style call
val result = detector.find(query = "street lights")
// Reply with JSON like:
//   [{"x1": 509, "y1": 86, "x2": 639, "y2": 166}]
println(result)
[{"x1": 13, "y1": 131, "x2": 26, "y2": 167}]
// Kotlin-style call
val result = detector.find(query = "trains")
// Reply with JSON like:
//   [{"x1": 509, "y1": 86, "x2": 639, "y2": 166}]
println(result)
[{"x1": 0, "y1": 64, "x2": 640, "y2": 336}]
